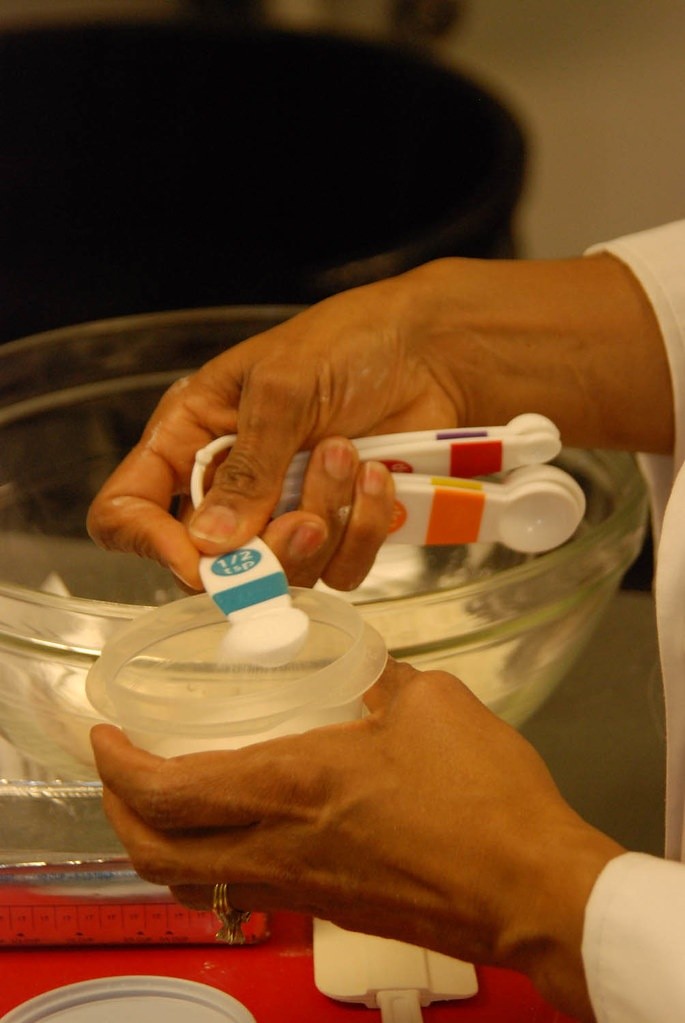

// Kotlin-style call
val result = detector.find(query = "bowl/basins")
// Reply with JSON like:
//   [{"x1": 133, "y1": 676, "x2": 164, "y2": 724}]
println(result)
[{"x1": 0, "y1": 306, "x2": 649, "y2": 788}]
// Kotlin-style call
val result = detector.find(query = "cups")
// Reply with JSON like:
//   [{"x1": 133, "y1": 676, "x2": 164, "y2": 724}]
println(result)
[{"x1": 84, "y1": 587, "x2": 388, "y2": 758}]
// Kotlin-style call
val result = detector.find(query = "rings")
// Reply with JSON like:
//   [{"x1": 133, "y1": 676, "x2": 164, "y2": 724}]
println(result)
[{"x1": 213, "y1": 884, "x2": 252, "y2": 944}]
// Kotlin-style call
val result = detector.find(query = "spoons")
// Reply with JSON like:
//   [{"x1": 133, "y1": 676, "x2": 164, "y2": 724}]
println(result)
[
  {"x1": 191, "y1": 412, "x2": 587, "y2": 554},
  {"x1": 198, "y1": 536, "x2": 309, "y2": 669}
]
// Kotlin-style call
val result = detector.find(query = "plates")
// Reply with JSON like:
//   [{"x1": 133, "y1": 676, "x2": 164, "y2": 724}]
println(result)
[{"x1": 0, "y1": 975, "x2": 256, "y2": 1023}]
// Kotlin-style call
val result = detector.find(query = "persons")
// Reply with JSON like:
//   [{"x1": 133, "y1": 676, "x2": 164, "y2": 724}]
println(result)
[{"x1": 86, "y1": 221, "x2": 685, "y2": 1023}]
